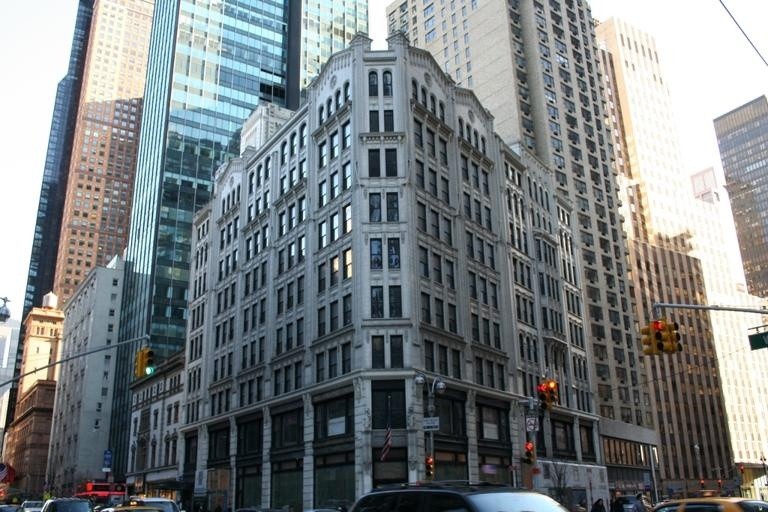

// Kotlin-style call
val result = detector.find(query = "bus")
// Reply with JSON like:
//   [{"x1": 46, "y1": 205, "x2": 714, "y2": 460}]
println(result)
[{"x1": 72, "y1": 481, "x2": 128, "y2": 502}]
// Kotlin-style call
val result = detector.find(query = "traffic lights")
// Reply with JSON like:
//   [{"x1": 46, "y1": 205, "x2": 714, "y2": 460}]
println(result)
[
  {"x1": 524, "y1": 441, "x2": 536, "y2": 467},
  {"x1": 538, "y1": 376, "x2": 559, "y2": 412},
  {"x1": 425, "y1": 456, "x2": 434, "y2": 479},
  {"x1": 740, "y1": 464, "x2": 745, "y2": 474},
  {"x1": 640, "y1": 319, "x2": 683, "y2": 356},
  {"x1": 133, "y1": 346, "x2": 155, "y2": 378},
  {"x1": 717, "y1": 479, "x2": 722, "y2": 490},
  {"x1": 699, "y1": 480, "x2": 706, "y2": 490}
]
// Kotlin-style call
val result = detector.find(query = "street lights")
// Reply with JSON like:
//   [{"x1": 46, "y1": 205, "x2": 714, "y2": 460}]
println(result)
[{"x1": 414, "y1": 369, "x2": 446, "y2": 457}]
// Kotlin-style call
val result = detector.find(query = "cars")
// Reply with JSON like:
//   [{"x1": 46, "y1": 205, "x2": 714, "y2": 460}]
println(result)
[
  {"x1": 94, "y1": 497, "x2": 188, "y2": 512},
  {"x1": 347, "y1": 479, "x2": 571, "y2": 512},
  {"x1": 650, "y1": 497, "x2": 768, "y2": 512},
  {"x1": 610, "y1": 494, "x2": 655, "y2": 512}
]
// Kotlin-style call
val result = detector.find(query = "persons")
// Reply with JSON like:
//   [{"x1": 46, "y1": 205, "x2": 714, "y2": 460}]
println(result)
[
  {"x1": 590, "y1": 497, "x2": 606, "y2": 512},
  {"x1": 575, "y1": 499, "x2": 587, "y2": 512},
  {"x1": 610, "y1": 498, "x2": 625, "y2": 511},
  {"x1": 631, "y1": 492, "x2": 651, "y2": 511}
]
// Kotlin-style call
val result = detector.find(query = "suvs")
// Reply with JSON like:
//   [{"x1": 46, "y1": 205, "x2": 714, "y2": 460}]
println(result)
[{"x1": 0, "y1": 497, "x2": 94, "y2": 512}]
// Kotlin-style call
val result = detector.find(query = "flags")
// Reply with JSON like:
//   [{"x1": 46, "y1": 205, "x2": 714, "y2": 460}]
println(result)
[{"x1": 381, "y1": 424, "x2": 392, "y2": 461}]
